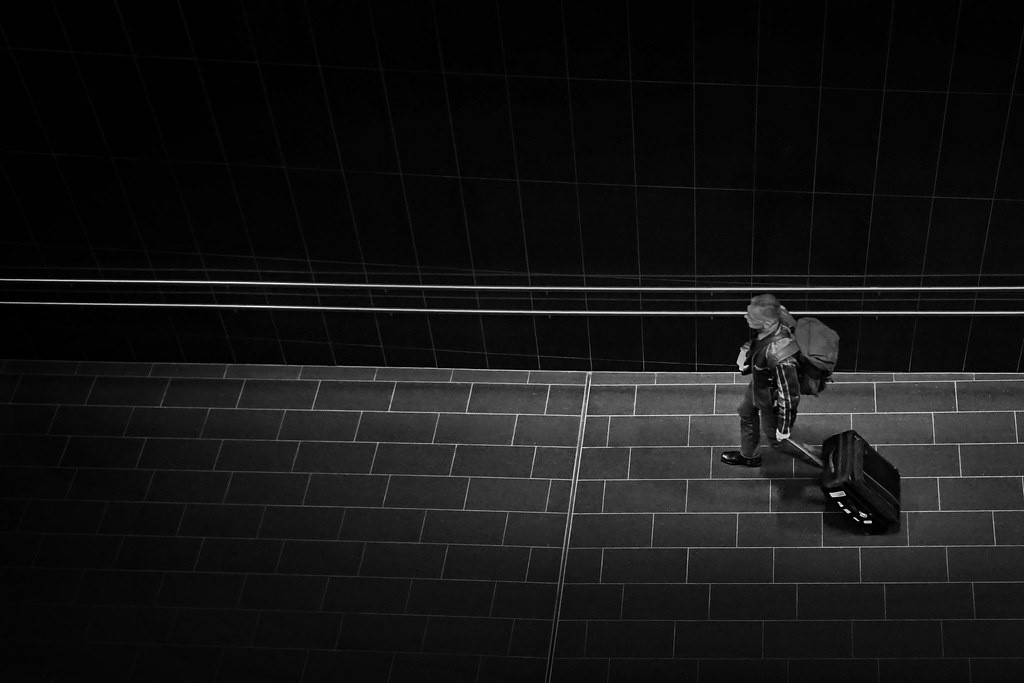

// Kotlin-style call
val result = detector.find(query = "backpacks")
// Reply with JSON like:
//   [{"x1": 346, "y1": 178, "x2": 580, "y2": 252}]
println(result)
[{"x1": 768, "y1": 317, "x2": 839, "y2": 395}]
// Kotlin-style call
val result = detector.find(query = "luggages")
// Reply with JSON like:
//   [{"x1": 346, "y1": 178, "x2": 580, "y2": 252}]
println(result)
[{"x1": 784, "y1": 430, "x2": 902, "y2": 535}]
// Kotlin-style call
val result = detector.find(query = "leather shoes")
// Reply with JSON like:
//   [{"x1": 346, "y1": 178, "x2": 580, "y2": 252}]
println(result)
[{"x1": 721, "y1": 451, "x2": 762, "y2": 468}]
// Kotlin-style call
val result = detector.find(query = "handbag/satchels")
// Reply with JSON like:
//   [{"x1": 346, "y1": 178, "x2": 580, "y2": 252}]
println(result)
[{"x1": 742, "y1": 350, "x2": 752, "y2": 376}]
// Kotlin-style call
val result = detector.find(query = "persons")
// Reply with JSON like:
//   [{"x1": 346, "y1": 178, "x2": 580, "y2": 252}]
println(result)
[{"x1": 717, "y1": 293, "x2": 803, "y2": 468}]
